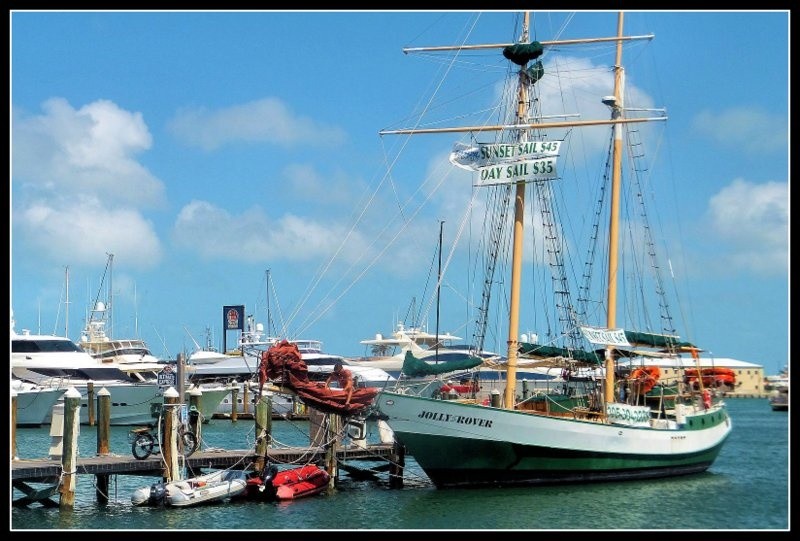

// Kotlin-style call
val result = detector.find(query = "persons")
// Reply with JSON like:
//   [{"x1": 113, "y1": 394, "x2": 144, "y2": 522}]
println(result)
[{"x1": 324, "y1": 363, "x2": 356, "y2": 405}]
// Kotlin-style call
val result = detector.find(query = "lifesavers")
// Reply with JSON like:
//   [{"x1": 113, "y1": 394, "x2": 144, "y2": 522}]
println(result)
[
  {"x1": 703, "y1": 390, "x2": 711, "y2": 408},
  {"x1": 631, "y1": 367, "x2": 660, "y2": 394},
  {"x1": 563, "y1": 368, "x2": 570, "y2": 380},
  {"x1": 685, "y1": 368, "x2": 735, "y2": 376},
  {"x1": 684, "y1": 376, "x2": 736, "y2": 385}
]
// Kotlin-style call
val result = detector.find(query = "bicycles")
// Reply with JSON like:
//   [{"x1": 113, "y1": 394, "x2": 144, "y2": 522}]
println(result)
[{"x1": 130, "y1": 412, "x2": 198, "y2": 460}]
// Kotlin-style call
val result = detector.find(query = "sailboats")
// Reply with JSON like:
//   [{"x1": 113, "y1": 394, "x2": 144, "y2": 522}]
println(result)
[{"x1": 373, "y1": 9, "x2": 735, "y2": 487}]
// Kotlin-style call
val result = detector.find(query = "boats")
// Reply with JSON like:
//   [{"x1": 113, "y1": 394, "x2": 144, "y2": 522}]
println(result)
[
  {"x1": 244, "y1": 464, "x2": 331, "y2": 501},
  {"x1": 683, "y1": 366, "x2": 735, "y2": 393},
  {"x1": 9, "y1": 264, "x2": 240, "y2": 424},
  {"x1": 763, "y1": 364, "x2": 789, "y2": 412},
  {"x1": 130, "y1": 467, "x2": 247, "y2": 507},
  {"x1": 69, "y1": 253, "x2": 462, "y2": 420}
]
